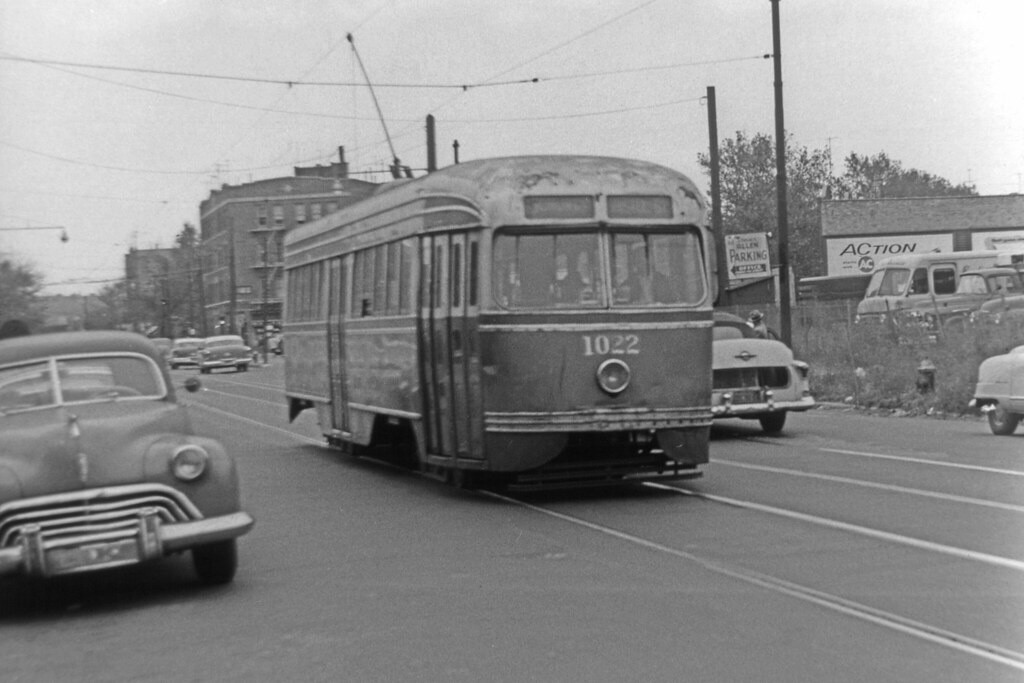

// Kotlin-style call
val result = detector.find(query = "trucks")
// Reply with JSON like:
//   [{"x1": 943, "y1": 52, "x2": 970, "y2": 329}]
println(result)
[
  {"x1": 908, "y1": 266, "x2": 1024, "y2": 344},
  {"x1": 969, "y1": 292, "x2": 1023, "y2": 342}
]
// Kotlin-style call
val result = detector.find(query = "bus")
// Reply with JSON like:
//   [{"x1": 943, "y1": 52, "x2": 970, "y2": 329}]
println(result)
[
  {"x1": 280, "y1": 154, "x2": 720, "y2": 495},
  {"x1": 796, "y1": 274, "x2": 871, "y2": 326}
]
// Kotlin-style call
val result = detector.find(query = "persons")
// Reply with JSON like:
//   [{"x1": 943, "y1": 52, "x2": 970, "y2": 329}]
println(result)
[
  {"x1": 255, "y1": 334, "x2": 268, "y2": 364},
  {"x1": 548, "y1": 254, "x2": 585, "y2": 304},
  {"x1": 748, "y1": 310, "x2": 767, "y2": 339},
  {"x1": 618, "y1": 243, "x2": 677, "y2": 305}
]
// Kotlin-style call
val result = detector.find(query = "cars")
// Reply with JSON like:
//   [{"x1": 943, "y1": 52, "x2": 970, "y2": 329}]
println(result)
[
  {"x1": 198, "y1": 334, "x2": 255, "y2": 372},
  {"x1": 270, "y1": 333, "x2": 281, "y2": 355},
  {"x1": 712, "y1": 310, "x2": 815, "y2": 437},
  {"x1": 971, "y1": 342, "x2": 1023, "y2": 435},
  {"x1": 168, "y1": 335, "x2": 205, "y2": 371},
  {"x1": 0, "y1": 331, "x2": 253, "y2": 592},
  {"x1": 152, "y1": 339, "x2": 172, "y2": 367}
]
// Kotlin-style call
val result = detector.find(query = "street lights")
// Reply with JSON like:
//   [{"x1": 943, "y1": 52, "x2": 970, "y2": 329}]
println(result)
[{"x1": 0, "y1": 225, "x2": 68, "y2": 241}]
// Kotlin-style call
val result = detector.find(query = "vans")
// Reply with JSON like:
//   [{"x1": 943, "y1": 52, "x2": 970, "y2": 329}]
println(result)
[{"x1": 854, "y1": 251, "x2": 1011, "y2": 322}]
